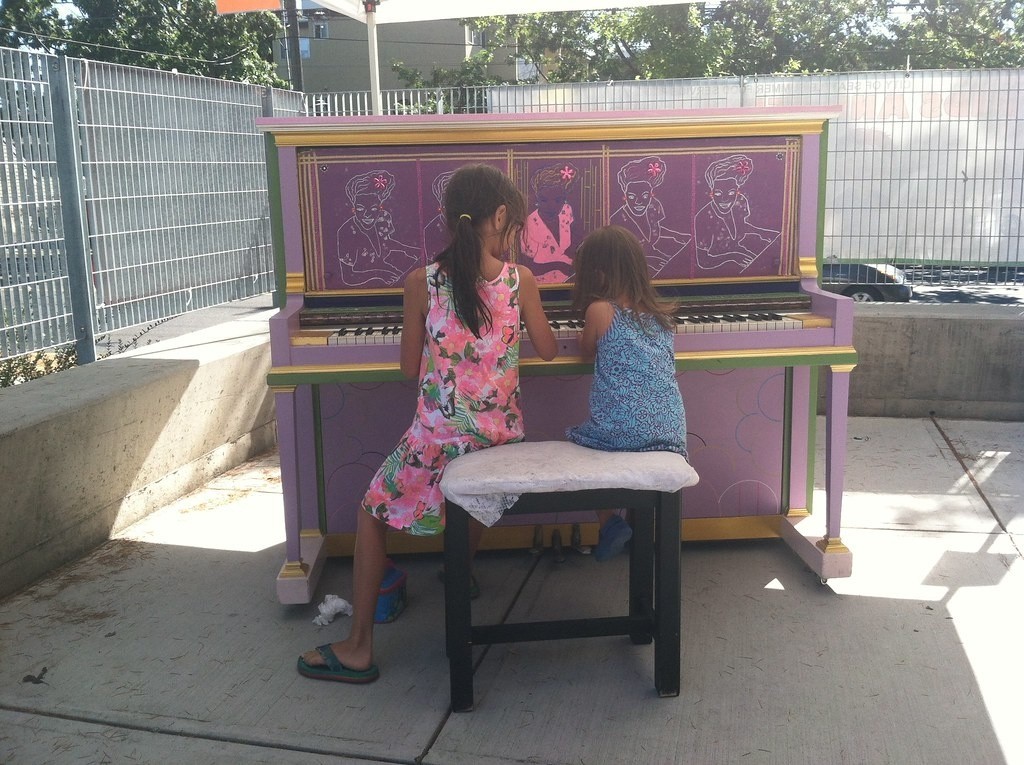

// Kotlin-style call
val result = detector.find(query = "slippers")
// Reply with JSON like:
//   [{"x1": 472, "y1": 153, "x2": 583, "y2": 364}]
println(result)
[
  {"x1": 298, "y1": 642, "x2": 378, "y2": 683},
  {"x1": 438, "y1": 567, "x2": 479, "y2": 596}
]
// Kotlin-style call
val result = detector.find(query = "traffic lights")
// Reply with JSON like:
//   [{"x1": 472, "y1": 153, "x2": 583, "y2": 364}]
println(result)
[{"x1": 365, "y1": 0, "x2": 376, "y2": 12}]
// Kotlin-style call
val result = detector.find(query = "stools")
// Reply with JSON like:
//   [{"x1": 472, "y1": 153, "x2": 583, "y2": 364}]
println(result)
[{"x1": 438, "y1": 441, "x2": 697, "y2": 713}]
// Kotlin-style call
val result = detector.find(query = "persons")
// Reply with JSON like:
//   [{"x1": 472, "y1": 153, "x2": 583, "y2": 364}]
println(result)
[
  {"x1": 292, "y1": 161, "x2": 558, "y2": 683},
  {"x1": 565, "y1": 225, "x2": 692, "y2": 559}
]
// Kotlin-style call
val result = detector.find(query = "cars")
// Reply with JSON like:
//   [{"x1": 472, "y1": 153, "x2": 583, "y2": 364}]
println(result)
[{"x1": 821, "y1": 263, "x2": 915, "y2": 303}]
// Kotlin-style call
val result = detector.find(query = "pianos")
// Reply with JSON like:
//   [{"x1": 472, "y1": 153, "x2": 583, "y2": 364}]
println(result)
[{"x1": 257, "y1": 103, "x2": 856, "y2": 604}]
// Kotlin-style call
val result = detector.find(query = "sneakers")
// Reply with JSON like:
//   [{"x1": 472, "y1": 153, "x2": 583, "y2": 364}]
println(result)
[{"x1": 590, "y1": 517, "x2": 631, "y2": 562}]
